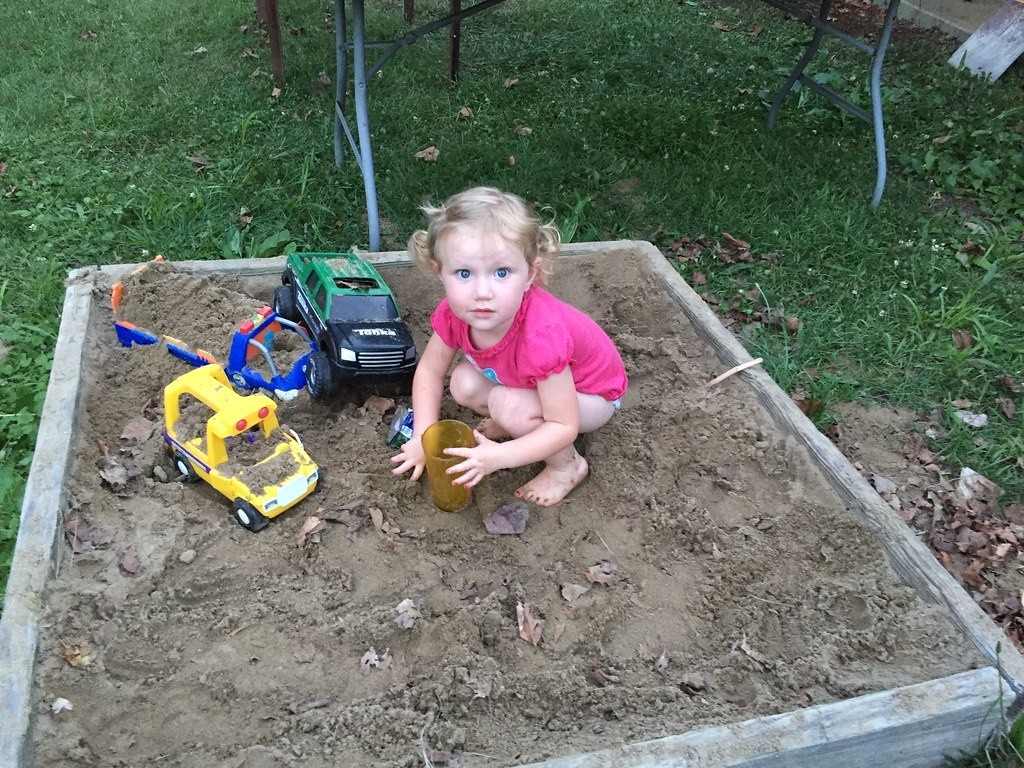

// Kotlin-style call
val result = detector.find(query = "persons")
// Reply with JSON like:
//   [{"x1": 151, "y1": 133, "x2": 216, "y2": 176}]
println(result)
[{"x1": 390, "y1": 187, "x2": 628, "y2": 507}]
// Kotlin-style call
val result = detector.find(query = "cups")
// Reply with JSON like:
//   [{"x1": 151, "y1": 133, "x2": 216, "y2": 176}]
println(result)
[{"x1": 421, "y1": 419, "x2": 478, "y2": 512}]
